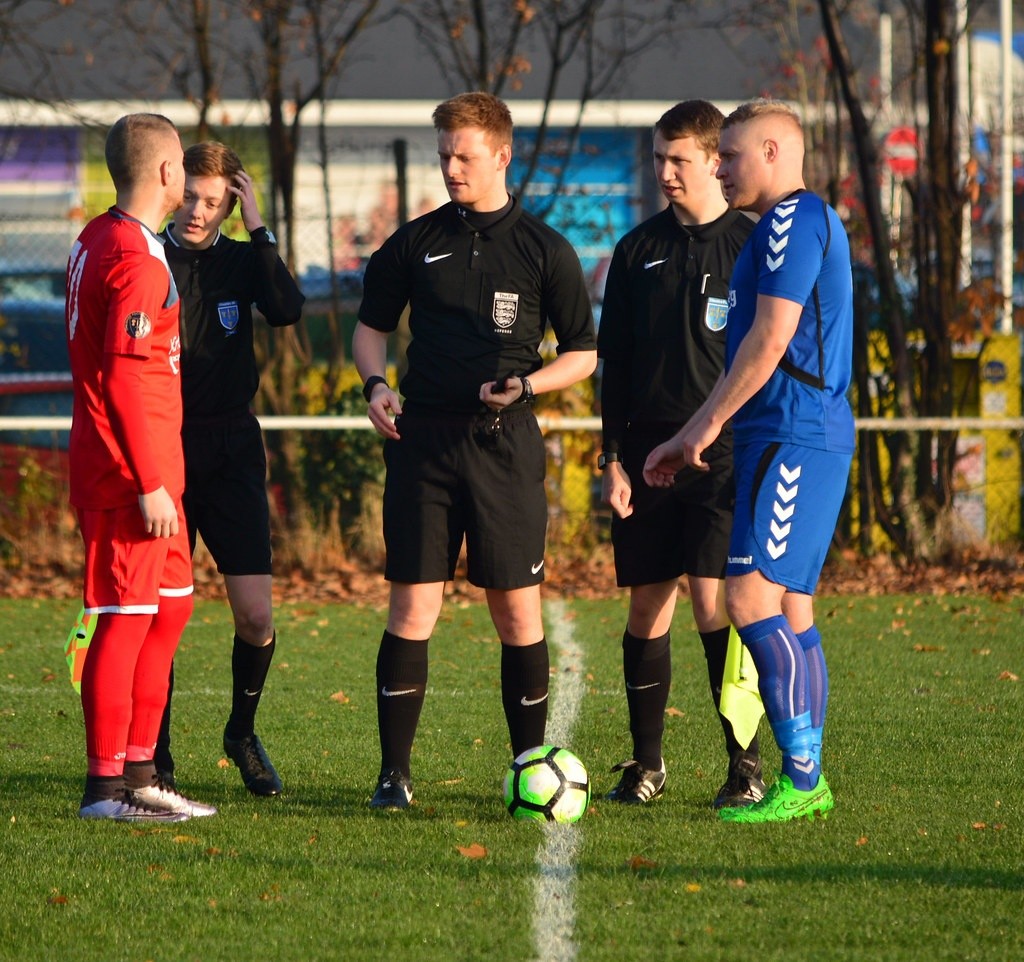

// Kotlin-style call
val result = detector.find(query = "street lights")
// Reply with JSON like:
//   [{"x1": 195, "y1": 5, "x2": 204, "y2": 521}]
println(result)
[{"x1": 390, "y1": 135, "x2": 410, "y2": 228}]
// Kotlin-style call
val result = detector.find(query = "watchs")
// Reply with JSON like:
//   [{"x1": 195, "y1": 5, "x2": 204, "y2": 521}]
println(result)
[
  {"x1": 263, "y1": 231, "x2": 277, "y2": 245},
  {"x1": 598, "y1": 451, "x2": 623, "y2": 470},
  {"x1": 515, "y1": 377, "x2": 533, "y2": 403}
]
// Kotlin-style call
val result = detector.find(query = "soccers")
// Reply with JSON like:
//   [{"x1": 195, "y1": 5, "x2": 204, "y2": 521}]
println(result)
[{"x1": 503, "y1": 744, "x2": 592, "y2": 824}]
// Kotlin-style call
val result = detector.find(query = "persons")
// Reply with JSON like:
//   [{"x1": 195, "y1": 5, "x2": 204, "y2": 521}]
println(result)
[
  {"x1": 597, "y1": 100, "x2": 764, "y2": 809},
  {"x1": 642, "y1": 102, "x2": 855, "y2": 822},
  {"x1": 156, "y1": 141, "x2": 307, "y2": 797},
  {"x1": 351, "y1": 92, "x2": 598, "y2": 813},
  {"x1": 65, "y1": 113, "x2": 217, "y2": 824}
]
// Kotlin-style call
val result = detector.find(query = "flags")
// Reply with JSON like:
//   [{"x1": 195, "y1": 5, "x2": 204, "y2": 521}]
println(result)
[
  {"x1": 719, "y1": 623, "x2": 766, "y2": 751},
  {"x1": 63, "y1": 605, "x2": 98, "y2": 694}
]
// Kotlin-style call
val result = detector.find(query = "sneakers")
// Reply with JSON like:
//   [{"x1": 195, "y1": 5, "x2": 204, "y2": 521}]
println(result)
[
  {"x1": 78, "y1": 790, "x2": 190, "y2": 826},
  {"x1": 156, "y1": 754, "x2": 180, "y2": 791},
  {"x1": 367, "y1": 768, "x2": 415, "y2": 812},
  {"x1": 220, "y1": 728, "x2": 286, "y2": 799},
  {"x1": 602, "y1": 754, "x2": 672, "y2": 806},
  {"x1": 719, "y1": 772, "x2": 837, "y2": 826},
  {"x1": 713, "y1": 752, "x2": 765, "y2": 813},
  {"x1": 128, "y1": 775, "x2": 219, "y2": 819}
]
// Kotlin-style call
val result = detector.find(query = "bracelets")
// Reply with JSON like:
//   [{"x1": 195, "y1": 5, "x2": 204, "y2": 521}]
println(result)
[{"x1": 362, "y1": 376, "x2": 389, "y2": 403}]
